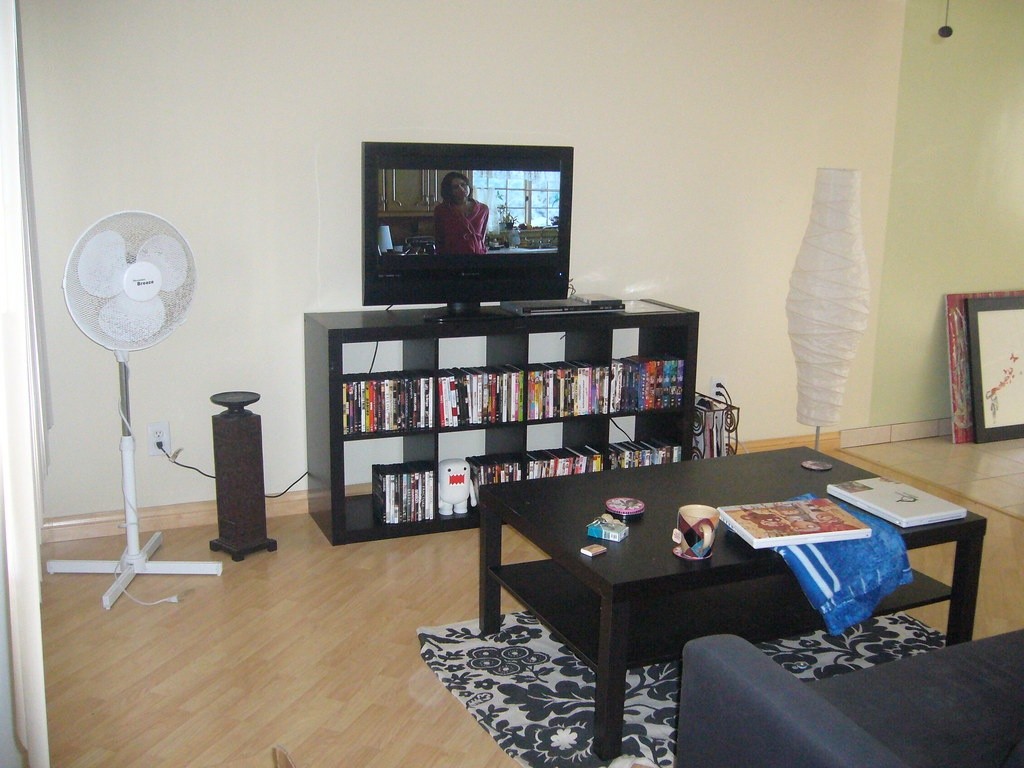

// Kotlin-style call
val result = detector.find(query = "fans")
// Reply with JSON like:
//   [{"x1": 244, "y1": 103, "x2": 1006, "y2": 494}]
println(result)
[{"x1": 43, "y1": 210, "x2": 222, "y2": 609}]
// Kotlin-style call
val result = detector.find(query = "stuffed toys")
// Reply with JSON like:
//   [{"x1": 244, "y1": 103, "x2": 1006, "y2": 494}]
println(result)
[{"x1": 438, "y1": 459, "x2": 477, "y2": 515}]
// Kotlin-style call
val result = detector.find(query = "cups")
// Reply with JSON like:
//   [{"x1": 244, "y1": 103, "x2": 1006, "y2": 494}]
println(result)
[{"x1": 679, "y1": 504, "x2": 719, "y2": 558}]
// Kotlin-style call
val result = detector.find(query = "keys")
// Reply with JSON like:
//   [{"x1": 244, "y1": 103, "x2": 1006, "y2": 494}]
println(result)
[{"x1": 586, "y1": 514, "x2": 627, "y2": 529}]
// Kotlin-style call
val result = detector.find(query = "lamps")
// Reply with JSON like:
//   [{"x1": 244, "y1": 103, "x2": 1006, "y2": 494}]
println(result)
[{"x1": 785, "y1": 169, "x2": 866, "y2": 469}]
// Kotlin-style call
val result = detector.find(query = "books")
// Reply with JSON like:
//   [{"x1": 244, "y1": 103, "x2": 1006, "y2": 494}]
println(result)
[
  {"x1": 826, "y1": 478, "x2": 966, "y2": 528},
  {"x1": 465, "y1": 440, "x2": 682, "y2": 508},
  {"x1": 372, "y1": 460, "x2": 434, "y2": 524},
  {"x1": 717, "y1": 498, "x2": 872, "y2": 549},
  {"x1": 343, "y1": 356, "x2": 685, "y2": 435}
]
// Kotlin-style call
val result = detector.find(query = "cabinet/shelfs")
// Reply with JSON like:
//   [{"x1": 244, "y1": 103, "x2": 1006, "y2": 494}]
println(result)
[{"x1": 304, "y1": 298, "x2": 701, "y2": 546}]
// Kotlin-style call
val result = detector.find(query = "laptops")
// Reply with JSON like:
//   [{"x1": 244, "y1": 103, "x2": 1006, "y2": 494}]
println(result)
[{"x1": 826, "y1": 476, "x2": 967, "y2": 528}]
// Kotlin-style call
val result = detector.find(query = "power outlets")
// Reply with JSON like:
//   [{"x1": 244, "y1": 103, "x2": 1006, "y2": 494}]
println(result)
[
  {"x1": 147, "y1": 422, "x2": 170, "y2": 456},
  {"x1": 711, "y1": 376, "x2": 726, "y2": 400}
]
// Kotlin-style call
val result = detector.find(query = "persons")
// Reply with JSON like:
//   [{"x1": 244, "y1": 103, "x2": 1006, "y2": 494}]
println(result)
[{"x1": 434, "y1": 172, "x2": 489, "y2": 255}]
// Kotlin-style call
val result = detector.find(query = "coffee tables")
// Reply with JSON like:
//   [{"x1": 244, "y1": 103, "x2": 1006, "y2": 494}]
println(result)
[{"x1": 474, "y1": 448, "x2": 986, "y2": 759}]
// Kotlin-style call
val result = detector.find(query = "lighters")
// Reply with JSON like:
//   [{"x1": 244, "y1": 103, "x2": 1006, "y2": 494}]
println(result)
[{"x1": 580, "y1": 544, "x2": 607, "y2": 557}]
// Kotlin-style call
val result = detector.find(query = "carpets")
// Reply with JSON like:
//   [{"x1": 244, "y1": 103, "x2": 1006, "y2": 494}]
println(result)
[{"x1": 417, "y1": 611, "x2": 946, "y2": 768}]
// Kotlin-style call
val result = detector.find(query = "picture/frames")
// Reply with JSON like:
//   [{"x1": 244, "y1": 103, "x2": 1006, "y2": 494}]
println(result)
[{"x1": 966, "y1": 297, "x2": 1024, "y2": 444}]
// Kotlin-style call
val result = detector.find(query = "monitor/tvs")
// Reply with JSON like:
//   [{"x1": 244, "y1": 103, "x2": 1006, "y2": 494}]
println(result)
[{"x1": 361, "y1": 141, "x2": 573, "y2": 321}]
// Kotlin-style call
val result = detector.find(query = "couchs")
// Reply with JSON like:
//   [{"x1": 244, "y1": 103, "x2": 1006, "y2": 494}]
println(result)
[{"x1": 676, "y1": 629, "x2": 1024, "y2": 768}]
